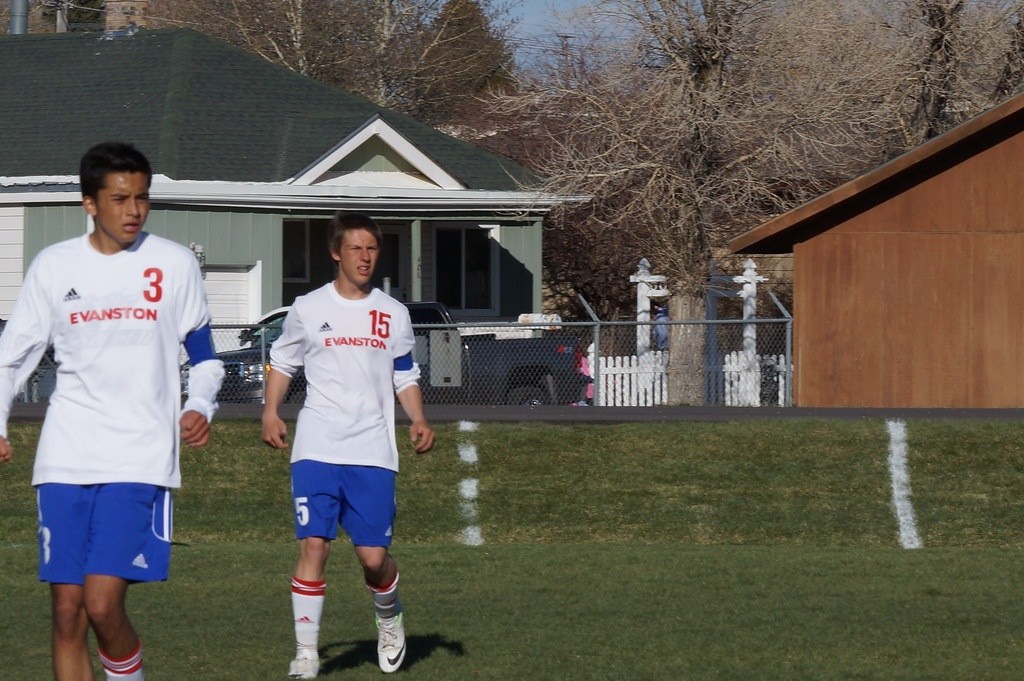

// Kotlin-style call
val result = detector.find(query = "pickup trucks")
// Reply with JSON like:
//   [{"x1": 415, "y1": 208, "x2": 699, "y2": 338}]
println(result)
[{"x1": 179, "y1": 299, "x2": 595, "y2": 404}]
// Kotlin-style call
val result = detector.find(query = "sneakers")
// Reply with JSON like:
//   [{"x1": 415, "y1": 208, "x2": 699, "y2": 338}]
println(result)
[
  {"x1": 375, "y1": 610, "x2": 406, "y2": 673},
  {"x1": 288, "y1": 647, "x2": 320, "y2": 679}
]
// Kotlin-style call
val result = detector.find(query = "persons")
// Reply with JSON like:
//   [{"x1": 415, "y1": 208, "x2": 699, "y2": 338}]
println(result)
[
  {"x1": 0, "y1": 141, "x2": 225, "y2": 681},
  {"x1": 567, "y1": 340, "x2": 594, "y2": 407},
  {"x1": 650, "y1": 299, "x2": 669, "y2": 352},
  {"x1": 262, "y1": 210, "x2": 434, "y2": 679}
]
